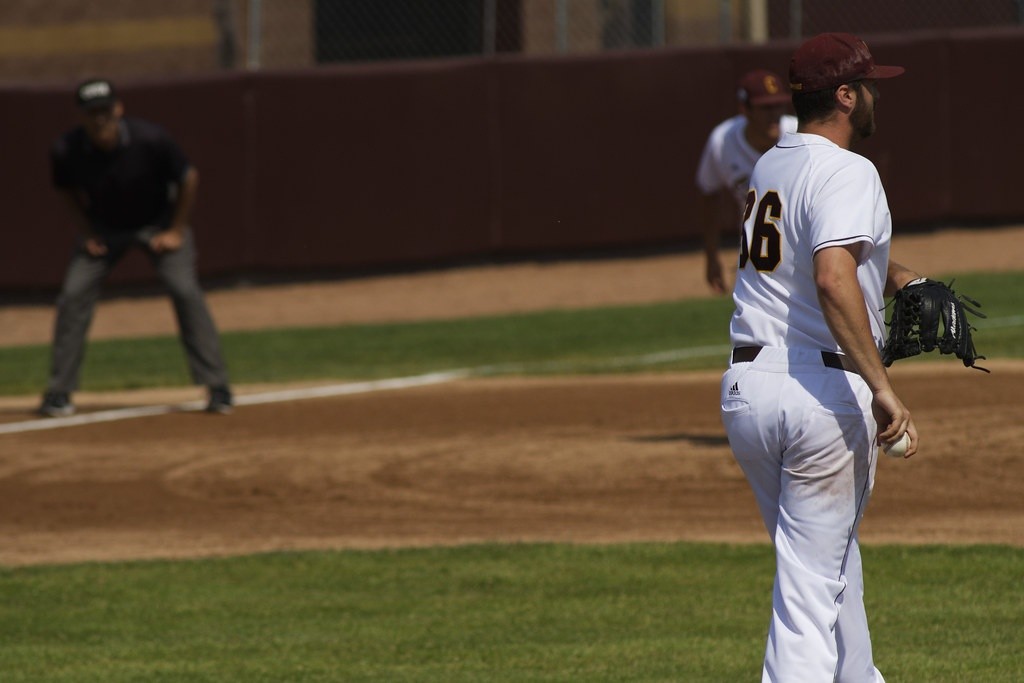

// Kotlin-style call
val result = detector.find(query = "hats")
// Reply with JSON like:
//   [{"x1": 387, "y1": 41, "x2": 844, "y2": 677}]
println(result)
[
  {"x1": 789, "y1": 34, "x2": 905, "y2": 93},
  {"x1": 745, "y1": 72, "x2": 795, "y2": 106},
  {"x1": 77, "y1": 79, "x2": 120, "y2": 113}
]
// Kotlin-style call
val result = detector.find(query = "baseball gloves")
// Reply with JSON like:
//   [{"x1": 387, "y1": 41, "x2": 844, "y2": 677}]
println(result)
[{"x1": 878, "y1": 277, "x2": 977, "y2": 369}]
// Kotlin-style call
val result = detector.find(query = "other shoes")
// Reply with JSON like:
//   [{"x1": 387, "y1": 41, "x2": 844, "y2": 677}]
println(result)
[
  {"x1": 40, "y1": 393, "x2": 74, "y2": 417},
  {"x1": 210, "y1": 387, "x2": 234, "y2": 414}
]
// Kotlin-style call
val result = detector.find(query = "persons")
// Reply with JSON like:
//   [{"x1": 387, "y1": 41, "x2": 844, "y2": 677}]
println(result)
[
  {"x1": 717, "y1": 30, "x2": 924, "y2": 683},
  {"x1": 691, "y1": 71, "x2": 798, "y2": 300},
  {"x1": 34, "y1": 78, "x2": 238, "y2": 420}
]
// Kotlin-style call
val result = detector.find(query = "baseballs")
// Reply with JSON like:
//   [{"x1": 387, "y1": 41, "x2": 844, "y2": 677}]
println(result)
[{"x1": 880, "y1": 431, "x2": 912, "y2": 457}]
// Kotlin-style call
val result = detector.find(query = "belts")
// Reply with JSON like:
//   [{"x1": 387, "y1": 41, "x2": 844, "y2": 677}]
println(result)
[{"x1": 732, "y1": 346, "x2": 860, "y2": 376}]
